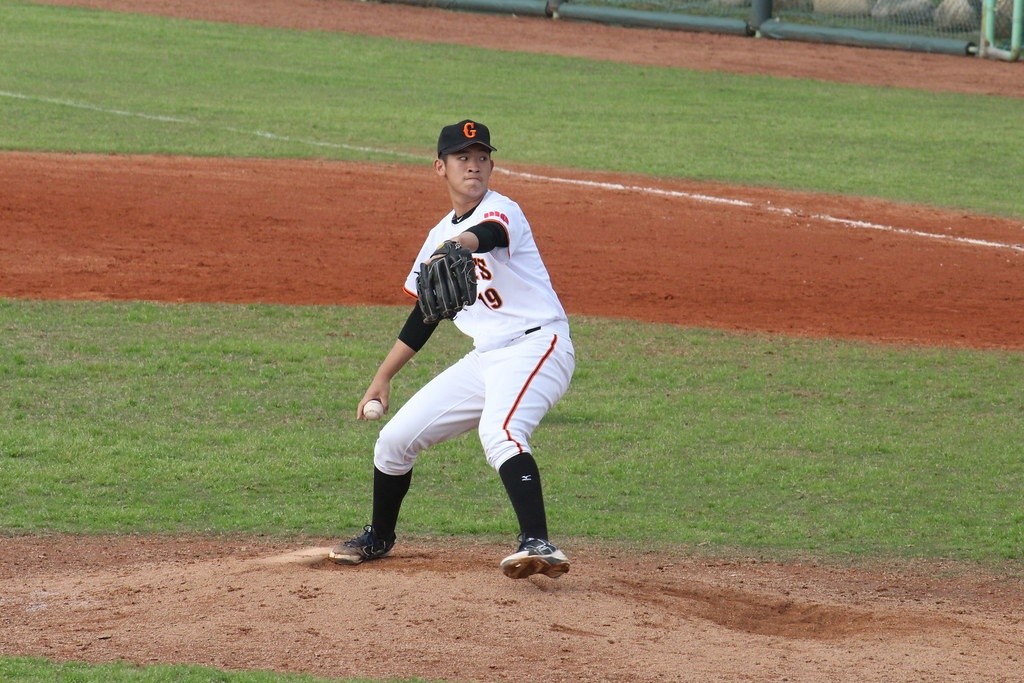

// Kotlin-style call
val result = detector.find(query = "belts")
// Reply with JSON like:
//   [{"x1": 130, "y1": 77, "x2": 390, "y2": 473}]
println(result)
[{"x1": 525, "y1": 326, "x2": 541, "y2": 334}]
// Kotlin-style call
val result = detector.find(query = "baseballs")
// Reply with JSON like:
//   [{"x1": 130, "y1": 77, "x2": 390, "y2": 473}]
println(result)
[{"x1": 363, "y1": 399, "x2": 384, "y2": 421}]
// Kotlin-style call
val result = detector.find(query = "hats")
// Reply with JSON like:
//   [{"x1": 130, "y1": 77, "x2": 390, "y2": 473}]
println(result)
[{"x1": 438, "y1": 120, "x2": 497, "y2": 158}]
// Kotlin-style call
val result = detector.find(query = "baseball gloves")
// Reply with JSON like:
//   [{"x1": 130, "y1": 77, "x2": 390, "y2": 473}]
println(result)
[{"x1": 416, "y1": 239, "x2": 478, "y2": 325}]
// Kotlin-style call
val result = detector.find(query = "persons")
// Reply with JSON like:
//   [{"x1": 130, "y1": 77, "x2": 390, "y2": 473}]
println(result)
[{"x1": 329, "y1": 119, "x2": 574, "y2": 579}]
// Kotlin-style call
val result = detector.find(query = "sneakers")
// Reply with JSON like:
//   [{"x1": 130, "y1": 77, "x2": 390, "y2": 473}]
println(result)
[
  {"x1": 500, "y1": 533, "x2": 570, "y2": 579},
  {"x1": 328, "y1": 525, "x2": 396, "y2": 565}
]
